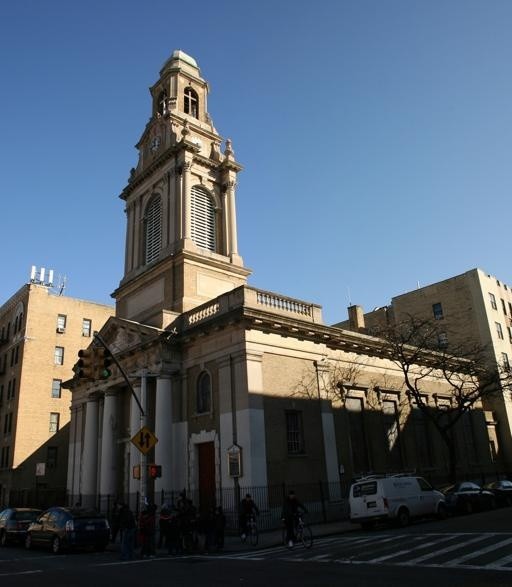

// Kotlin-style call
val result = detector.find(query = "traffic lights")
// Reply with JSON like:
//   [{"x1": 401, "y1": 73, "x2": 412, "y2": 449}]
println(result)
[
  {"x1": 98, "y1": 350, "x2": 111, "y2": 379},
  {"x1": 77, "y1": 350, "x2": 93, "y2": 379}
]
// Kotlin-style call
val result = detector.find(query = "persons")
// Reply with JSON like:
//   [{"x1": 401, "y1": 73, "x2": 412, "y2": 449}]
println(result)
[
  {"x1": 73, "y1": 501, "x2": 80, "y2": 508},
  {"x1": 211, "y1": 504, "x2": 227, "y2": 554},
  {"x1": 205, "y1": 505, "x2": 216, "y2": 531},
  {"x1": 281, "y1": 490, "x2": 309, "y2": 549},
  {"x1": 240, "y1": 494, "x2": 259, "y2": 541},
  {"x1": 107, "y1": 490, "x2": 215, "y2": 561}
]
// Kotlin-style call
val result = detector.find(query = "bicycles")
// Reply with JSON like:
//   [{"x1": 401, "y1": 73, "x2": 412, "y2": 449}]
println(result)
[
  {"x1": 237, "y1": 511, "x2": 262, "y2": 546},
  {"x1": 282, "y1": 510, "x2": 313, "y2": 550}
]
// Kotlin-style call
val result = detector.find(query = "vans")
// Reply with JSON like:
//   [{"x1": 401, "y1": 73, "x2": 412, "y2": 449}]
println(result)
[{"x1": 349, "y1": 474, "x2": 450, "y2": 526}]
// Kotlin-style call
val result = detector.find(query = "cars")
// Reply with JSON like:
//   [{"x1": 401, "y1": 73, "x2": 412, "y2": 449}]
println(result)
[
  {"x1": 441, "y1": 480, "x2": 512, "y2": 516},
  {"x1": 0, "y1": 506, "x2": 112, "y2": 555}
]
